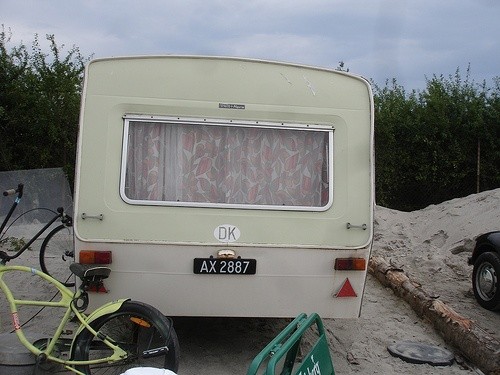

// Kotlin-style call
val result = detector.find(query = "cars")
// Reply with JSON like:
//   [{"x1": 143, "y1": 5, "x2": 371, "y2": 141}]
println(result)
[{"x1": 467, "y1": 230, "x2": 500, "y2": 311}]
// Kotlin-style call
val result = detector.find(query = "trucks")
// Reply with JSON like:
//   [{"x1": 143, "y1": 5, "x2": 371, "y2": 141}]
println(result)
[{"x1": 72, "y1": 54, "x2": 375, "y2": 320}]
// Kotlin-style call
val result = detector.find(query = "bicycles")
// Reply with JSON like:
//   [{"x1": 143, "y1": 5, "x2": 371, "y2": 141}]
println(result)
[
  {"x1": 0, "y1": 182, "x2": 181, "y2": 375},
  {"x1": 39, "y1": 222, "x2": 74, "y2": 287}
]
